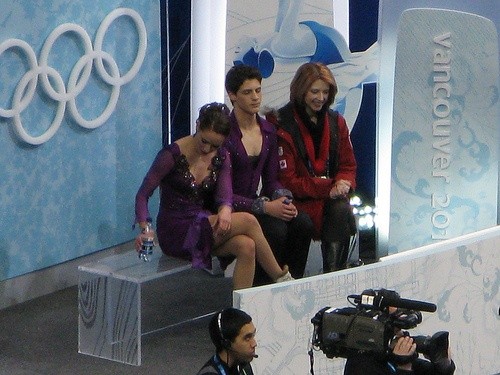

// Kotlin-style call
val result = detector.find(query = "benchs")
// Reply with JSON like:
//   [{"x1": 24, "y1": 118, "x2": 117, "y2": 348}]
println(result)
[{"x1": 77, "y1": 206, "x2": 360, "y2": 366}]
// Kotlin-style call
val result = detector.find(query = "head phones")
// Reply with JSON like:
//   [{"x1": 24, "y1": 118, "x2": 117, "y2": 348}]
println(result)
[{"x1": 217, "y1": 311, "x2": 231, "y2": 351}]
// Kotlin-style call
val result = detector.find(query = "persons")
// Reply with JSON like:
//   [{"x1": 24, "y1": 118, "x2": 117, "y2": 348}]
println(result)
[
  {"x1": 344, "y1": 290, "x2": 455, "y2": 374},
  {"x1": 265, "y1": 62, "x2": 358, "y2": 272},
  {"x1": 197, "y1": 307, "x2": 258, "y2": 375},
  {"x1": 136, "y1": 102, "x2": 297, "y2": 289},
  {"x1": 225, "y1": 64, "x2": 299, "y2": 286}
]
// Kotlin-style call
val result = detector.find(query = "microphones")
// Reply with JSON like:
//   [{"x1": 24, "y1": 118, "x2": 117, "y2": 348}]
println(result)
[
  {"x1": 348, "y1": 293, "x2": 437, "y2": 312},
  {"x1": 253, "y1": 354, "x2": 258, "y2": 358}
]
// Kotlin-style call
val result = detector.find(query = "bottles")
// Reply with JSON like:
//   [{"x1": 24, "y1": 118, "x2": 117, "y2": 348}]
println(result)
[{"x1": 139, "y1": 217, "x2": 155, "y2": 264}]
